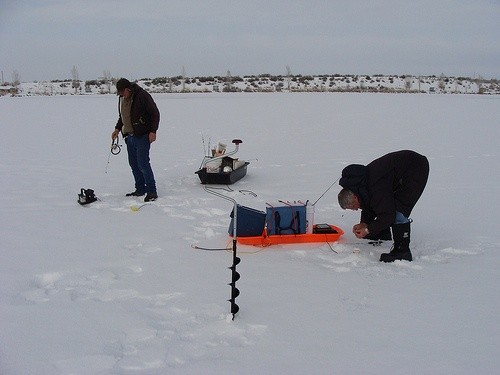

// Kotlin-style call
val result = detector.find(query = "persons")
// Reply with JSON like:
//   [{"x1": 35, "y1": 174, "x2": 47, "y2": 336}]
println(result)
[
  {"x1": 111, "y1": 78, "x2": 160, "y2": 203},
  {"x1": 336, "y1": 149, "x2": 430, "y2": 263}
]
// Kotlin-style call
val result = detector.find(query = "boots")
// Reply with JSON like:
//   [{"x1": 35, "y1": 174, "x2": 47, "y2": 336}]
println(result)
[
  {"x1": 379, "y1": 218, "x2": 412, "y2": 262},
  {"x1": 363, "y1": 218, "x2": 392, "y2": 240}
]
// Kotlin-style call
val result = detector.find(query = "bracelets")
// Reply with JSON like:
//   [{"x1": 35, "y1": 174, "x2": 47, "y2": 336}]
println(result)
[{"x1": 364, "y1": 227, "x2": 370, "y2": 235}]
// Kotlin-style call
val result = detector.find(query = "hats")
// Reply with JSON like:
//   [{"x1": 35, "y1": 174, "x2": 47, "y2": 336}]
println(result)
[{"x1": 116, "y1": 78, "x2": 129, "y2": 96}]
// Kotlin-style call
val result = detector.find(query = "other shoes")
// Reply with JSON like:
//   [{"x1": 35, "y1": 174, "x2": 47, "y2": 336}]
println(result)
[
  {"x1": 126, "y1": 189, "x2": 144, "y2": 196},
  {"x1": 144, "y1": 192, "x2": 158, "y2": 201}
]
